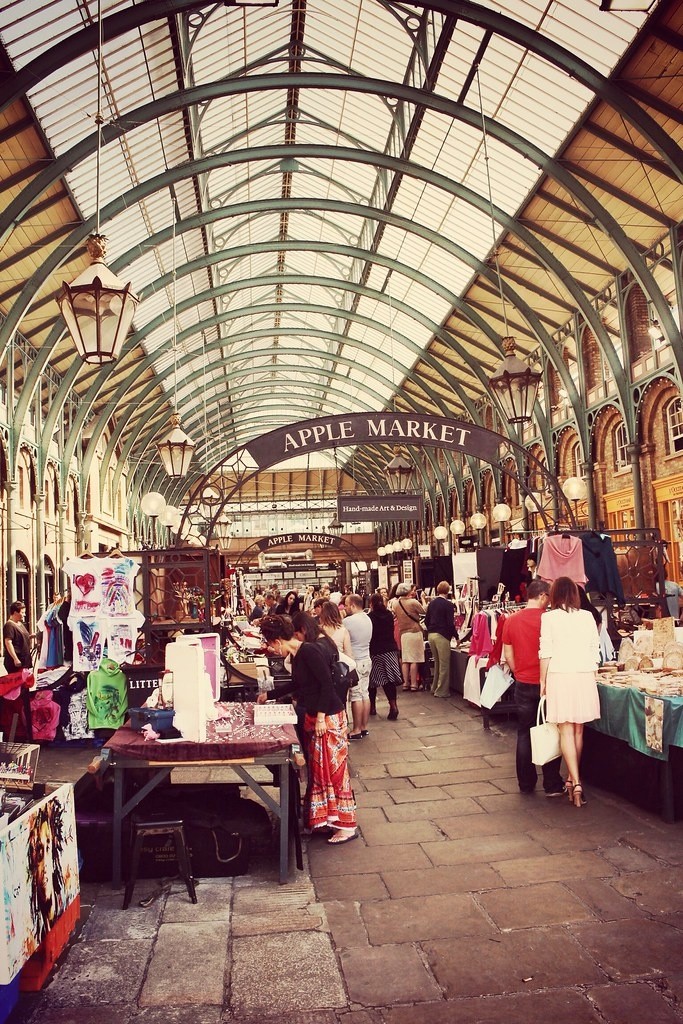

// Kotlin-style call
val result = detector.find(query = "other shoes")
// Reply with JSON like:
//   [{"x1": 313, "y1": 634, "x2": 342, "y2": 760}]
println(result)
[
  {"x1": 403, "y1": 683, "x2": 410, "y2": 691},
  {"x1": 411, "y1": 684, "x2": 419, "y2": 691},
  {"x1": 434, "y1": 692, "x2": 451, "y2": 698},
  {"x1": 361, "y1": 730, "x2": 369, "y2": 735},
  {"x1": 387, "y1": 705, "x2": 399, "y2": 720},
  {"x1": 370, "y1": 709, "x2": 377, "y2": 715},
  {"x1": 346, "y1": 733, "x2": 362, "y2": 741},
  {"x1": 546, "y1": 786, "x2": 567, "y2": 797}
]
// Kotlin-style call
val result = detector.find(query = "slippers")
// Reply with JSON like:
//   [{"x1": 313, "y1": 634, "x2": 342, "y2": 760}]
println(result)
[
  {"x1": 328, "y1": 829, "x2": 359, "y2": 844},
  {"x1": 313, "y1": 827, "x2": 333, "y2": 835}
]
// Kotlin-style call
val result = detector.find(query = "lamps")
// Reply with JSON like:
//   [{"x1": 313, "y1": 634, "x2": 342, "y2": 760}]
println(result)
[{"x1": 56, "y1": 1, "x2": 585, "y2": 556}]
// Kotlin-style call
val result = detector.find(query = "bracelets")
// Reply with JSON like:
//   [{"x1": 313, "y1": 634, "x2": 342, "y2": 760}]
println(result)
[{"x1": 317, "y1": 720, "x2": 325, "y2": 722}]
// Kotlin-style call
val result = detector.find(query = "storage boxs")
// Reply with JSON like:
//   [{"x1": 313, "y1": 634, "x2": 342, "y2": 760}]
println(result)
[{"x1": 206, "y1": 829, "x2": 249, "y2": 878}]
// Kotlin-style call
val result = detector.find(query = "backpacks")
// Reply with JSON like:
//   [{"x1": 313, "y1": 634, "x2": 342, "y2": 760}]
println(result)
[{"x1": 330, "y1": 653, "x2": 350, "y2": 693}]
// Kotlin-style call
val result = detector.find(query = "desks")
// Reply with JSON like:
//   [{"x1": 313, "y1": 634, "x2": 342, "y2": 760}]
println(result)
[
  {"x1": 449, "y1": 650, "x2": 522, "y2": 730},
  {"x1": 590, "y1": 681, "x2": 682, "y2": 823},
  {"x1": 1, "y1": 684, "x2": 305, "y2": 1024}
]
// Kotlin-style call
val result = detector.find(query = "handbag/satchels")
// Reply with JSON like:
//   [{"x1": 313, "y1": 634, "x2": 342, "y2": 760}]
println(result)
[
  {"x1": 530, "y1": 694, "x2": 563, "y2": 766},
  {"x1": 199, "y1": 826, "x2": 248, "y2": 876}
]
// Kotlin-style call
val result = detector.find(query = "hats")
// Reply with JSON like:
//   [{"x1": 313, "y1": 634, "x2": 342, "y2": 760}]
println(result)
[{"x1": 395, "y1": 584, "x2": 411, "y2": 595}]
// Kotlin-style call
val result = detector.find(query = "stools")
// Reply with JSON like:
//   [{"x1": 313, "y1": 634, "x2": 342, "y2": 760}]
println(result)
[{"x1": 122, "y1": 806, "x2": 198, "y2": 910}]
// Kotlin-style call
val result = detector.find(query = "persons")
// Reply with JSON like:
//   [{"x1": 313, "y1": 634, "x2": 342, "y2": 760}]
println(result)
[
  {"x1": 47, "y1": 593, "x2": 61, "y2": 609},
  {"x1": 250, "y1": 583, "x2": 428, "y2": 844},
  {"x1": 527, "y1": 553, "x2": 541, "y2": 586},
  {"x1": 502, "y1": 576, "x2": 603, "y2": 806},
  {"x1": 9, "y1": 799, "x2": 79, "y2": 976},
  {"x1": 654, "y1": 566, "x2": 683, "y2": 627},
  {"x1": 3, "y1": 601, "x2": 35, "y2": 674},
  {"x1": 424, "y1": 582, "x2": 461, "y2": 697}
]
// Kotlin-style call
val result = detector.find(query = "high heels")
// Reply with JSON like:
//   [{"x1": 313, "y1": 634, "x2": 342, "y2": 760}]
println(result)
[
  {"x1": 565, "y1": 780, "x2": 574, "y2": 802},
  {"x1": 573, "y1": 784, "x2": 588, "y2": 807}
]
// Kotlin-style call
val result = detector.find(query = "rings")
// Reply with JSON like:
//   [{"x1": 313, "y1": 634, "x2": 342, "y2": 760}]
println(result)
[{"x1": 207, "y1": 700, "x2": 293, "y2": 741}]
